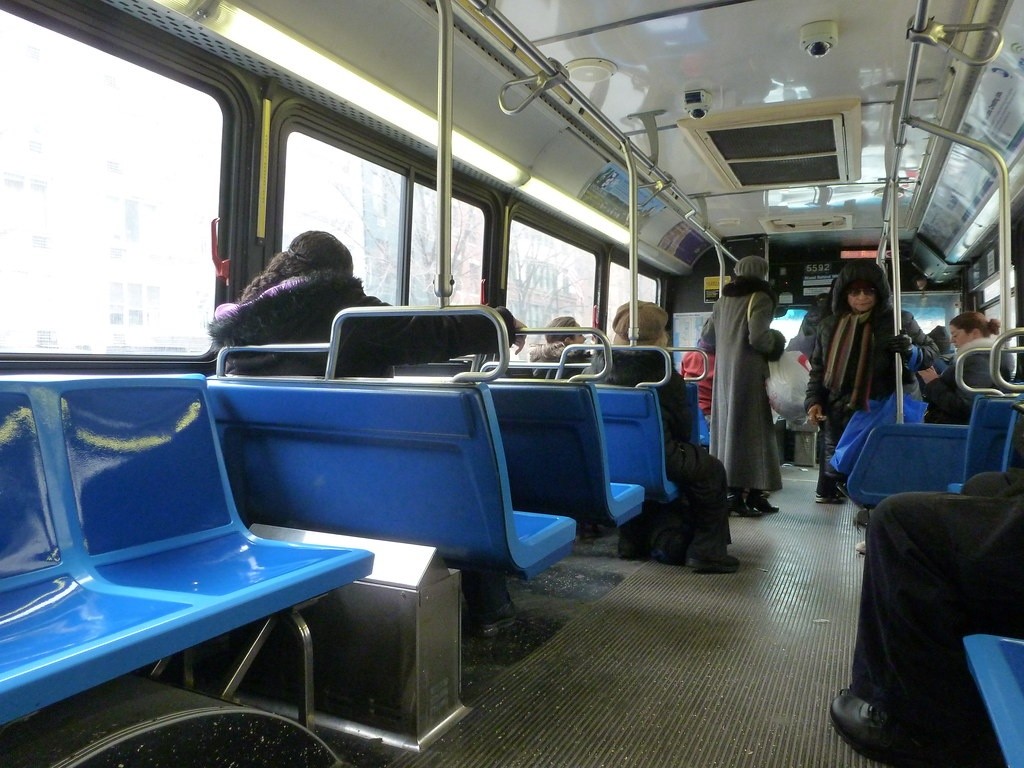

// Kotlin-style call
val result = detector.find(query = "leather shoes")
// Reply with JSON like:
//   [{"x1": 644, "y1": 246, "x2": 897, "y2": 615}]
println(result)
[{"x1": 830, "y1": 693, "x2": 961, "y2": 768}]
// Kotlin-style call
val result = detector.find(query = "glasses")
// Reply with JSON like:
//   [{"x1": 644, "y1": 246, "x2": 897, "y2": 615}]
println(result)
[{"x1": 848, "y1": 287, "x2": 876, "y2": 296}]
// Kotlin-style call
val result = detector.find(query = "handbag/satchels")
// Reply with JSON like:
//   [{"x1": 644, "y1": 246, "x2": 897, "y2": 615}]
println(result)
[
  {"x1": 831, "y1": 386, "x2": 929, "y2": 475},
  {"x1": 646, "y1": 518, "x2": 695, "y2": 565}
]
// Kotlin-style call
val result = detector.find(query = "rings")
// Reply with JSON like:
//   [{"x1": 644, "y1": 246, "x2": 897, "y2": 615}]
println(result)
[{"x1": 808, "y1": 416, "x2": 813, "y2": 419}]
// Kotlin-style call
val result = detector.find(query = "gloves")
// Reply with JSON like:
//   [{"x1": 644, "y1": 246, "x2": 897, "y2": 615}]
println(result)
[{"x1": 887, "y1": 329, "x2": 913, "y2": 355}]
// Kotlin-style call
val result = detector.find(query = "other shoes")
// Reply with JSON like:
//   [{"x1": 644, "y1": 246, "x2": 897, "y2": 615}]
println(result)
[
  {"x1": 583, "y1": 521, "x2": 603, "y2": 538},
  {"x1": 618, "y1": 538, "x2": 641, "y2": 558},
  {"x1": 816, "y1": 494, "x2": 848, "y2": 505},
  {"x1": 688, "y1": 555, "x2": 740, "y2": 574},
  {"x1": 475, "y1": 602, "x2": 528, "y2": 638}
]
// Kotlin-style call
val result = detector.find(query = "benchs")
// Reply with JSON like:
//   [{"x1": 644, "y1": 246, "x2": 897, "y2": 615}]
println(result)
[{"x1": 0, "y1": 307, "x2": 1024, "y2": 768}]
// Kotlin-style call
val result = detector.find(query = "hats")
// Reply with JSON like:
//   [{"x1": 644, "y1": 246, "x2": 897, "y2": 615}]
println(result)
[
  {"x1": 929, "y1": 326, "x2": 950, "y2": 354},
  {"x1": 734, "y1": 255, "x2": 768, "y2": 278},
  {"x1": 613, "y1": 301, "x2": 668, "y2": 341}
]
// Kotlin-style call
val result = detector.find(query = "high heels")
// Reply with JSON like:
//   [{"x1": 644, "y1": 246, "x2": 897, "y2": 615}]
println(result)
[
  {"x1": 746, "y1": 493, "x2": 780, "y2": 512},
  {"x1": 726, "y1": 488, "x2": 762, "y2": 517}
]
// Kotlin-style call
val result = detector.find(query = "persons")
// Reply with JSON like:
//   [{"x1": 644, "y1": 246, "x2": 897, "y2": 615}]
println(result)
[
  {"x1": 680, "y1": 338, "x2": 715, "y2": 432},
  {"x1": 829, "y1": 420, "x2": 1024, "y2": 768},
  {"x1": 784, "y1": 293, "x2": 832, "y2": 364},
  {"x1": 918, "y1": 311, "x2": 1016, "y2": 425},
  {"x1": 529, "y1": 317, "x2": 594, "y2": 379},
  {"x1": 206, "y1": 231, "x2": 527, "y2": 376},
  {"x1": 804, "y1": 259, "x2": 939, "y2": 504},
  {"x1": 927, "y1": 325, "x2": 950, "y2": 355},
  {"x1": 700, "y1": 255, "x2": 786, "y2": 517},
  {"x1": 580, "y1": 300, "x2": 740, "y2": 574}
]
